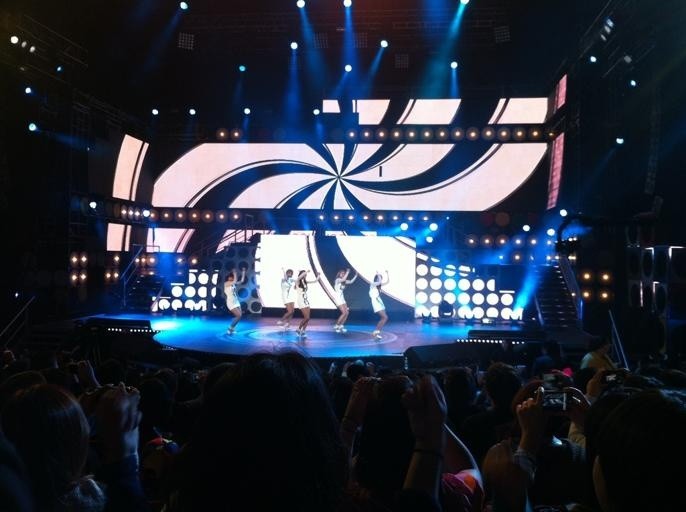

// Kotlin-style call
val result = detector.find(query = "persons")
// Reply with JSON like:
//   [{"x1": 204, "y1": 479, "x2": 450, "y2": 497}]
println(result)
[
  {"x1": 369, "y1": 270, "x2": 389, "y2": 339},
  {"x1": 334, "y1": 269, "x2": 358, "y2": 333},
  {"x1": 276, "y1": 266, "x2": 298, "y2": 326},
  {"x1": 295, "y1": 269, "x2": 321, "y2": 337},
  {"x1": 224, "y1": 267, "x2": 245, "y2": 334},
  {"x1": 2, "y1": 334, "x2": 685, "y2": 512}
]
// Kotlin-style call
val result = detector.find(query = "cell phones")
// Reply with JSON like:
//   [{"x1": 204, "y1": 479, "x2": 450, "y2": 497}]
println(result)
[
  {"x1": 541, "y1": 373, "x2": 557, "y2": 381},
  {"x1": 374, "y1": 380, "x2": 404, "y2": 402}
]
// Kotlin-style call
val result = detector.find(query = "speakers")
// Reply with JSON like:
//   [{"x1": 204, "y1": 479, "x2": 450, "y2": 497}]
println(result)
[
  {"x1": 92, "y1": 339, "x2": 125, "y2": 378},
  {"x1": 405, "y1": 340, "x2": 482, "y2": 371},
  {"x1": 56, "y1": 365, "x2": 89, "y2": 395},
  {"x1": 135, "y1": 344, "x2": 174, "y2": 366}
]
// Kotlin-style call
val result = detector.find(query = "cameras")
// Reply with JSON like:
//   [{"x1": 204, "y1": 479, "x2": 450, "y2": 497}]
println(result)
[
  {"x1": 534, "y1": 390, "x2": 572, "y2": 412},
  {"x1": 601, "y1": 372, "x2": 624, "y2": 384}
]
